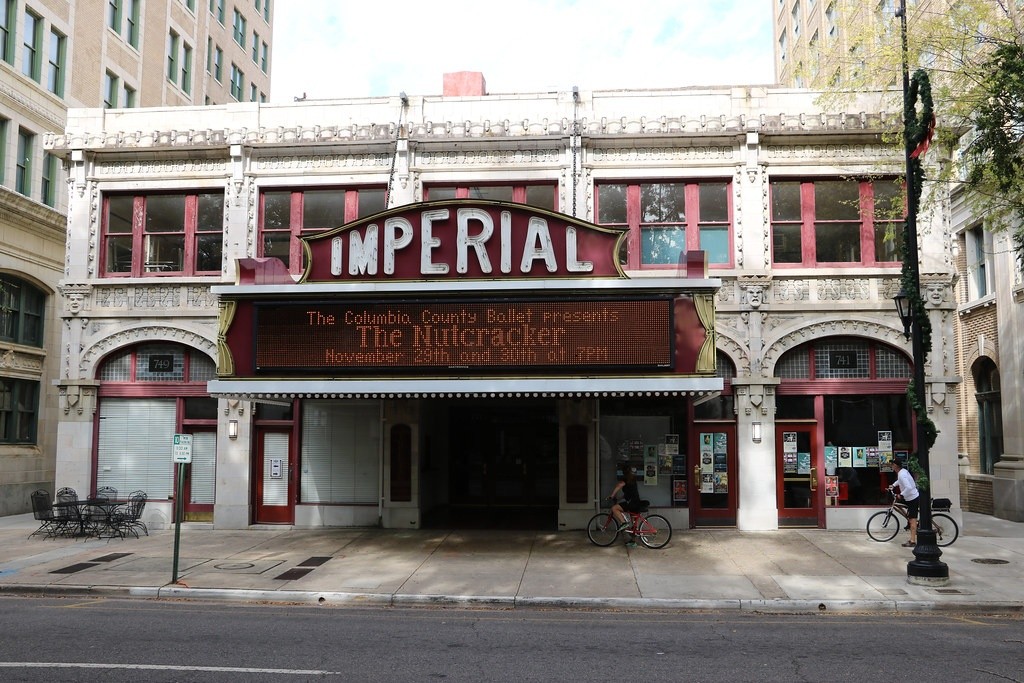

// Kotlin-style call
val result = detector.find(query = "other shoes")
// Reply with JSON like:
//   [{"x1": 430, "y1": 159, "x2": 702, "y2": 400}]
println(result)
[{"x1": 902, "y1": 540, "x2": 916, "y2": 547}]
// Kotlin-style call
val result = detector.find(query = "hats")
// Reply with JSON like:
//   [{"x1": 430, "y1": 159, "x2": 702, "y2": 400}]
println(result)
[{"x1": 889, "y1": 458, "x2": 903, "y2": 468}]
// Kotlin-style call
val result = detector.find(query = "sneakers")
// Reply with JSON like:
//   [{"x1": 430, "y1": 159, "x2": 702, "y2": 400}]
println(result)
[
  {"x1": 616, "y1": 521, "x2": 630, "y2": 532},
  {"x1": 625, "y1": 540, "x2": 638, "y2": 546}
]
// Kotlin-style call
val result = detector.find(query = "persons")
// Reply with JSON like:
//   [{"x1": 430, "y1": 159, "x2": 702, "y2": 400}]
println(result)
[
  {"x1": 889, "y1": 458, "x2": 919, "y2": 547},
  {"x1": 606, "y1": 463, "x2": 641, "y2": 547}
]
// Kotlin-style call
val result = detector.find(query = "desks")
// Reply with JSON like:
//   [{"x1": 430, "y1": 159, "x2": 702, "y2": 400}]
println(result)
[{"x1": 50, "y1": 499, "x2": 126, "y2": 537}]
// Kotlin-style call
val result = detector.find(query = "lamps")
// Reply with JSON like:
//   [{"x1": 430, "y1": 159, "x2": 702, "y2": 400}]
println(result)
[
  {"x1": 229, "y1": 420, "x2": 238, "y2": 438},
  {"x1": 752, "y1": 422, "x2": 762, "y2": 441}
]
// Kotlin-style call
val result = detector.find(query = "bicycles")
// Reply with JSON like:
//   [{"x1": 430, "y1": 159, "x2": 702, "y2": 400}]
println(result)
[
  {"x1": 587, "y1": 497, "x2": 672, "y2": 549},
  {"x1": 866, "y1": 488, "x2": 958, "y2": 547}
]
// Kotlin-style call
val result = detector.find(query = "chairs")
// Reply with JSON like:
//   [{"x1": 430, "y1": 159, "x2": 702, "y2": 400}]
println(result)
[{"x1": 27, "y1": 486, "x2": 148, "y2": 544}]
